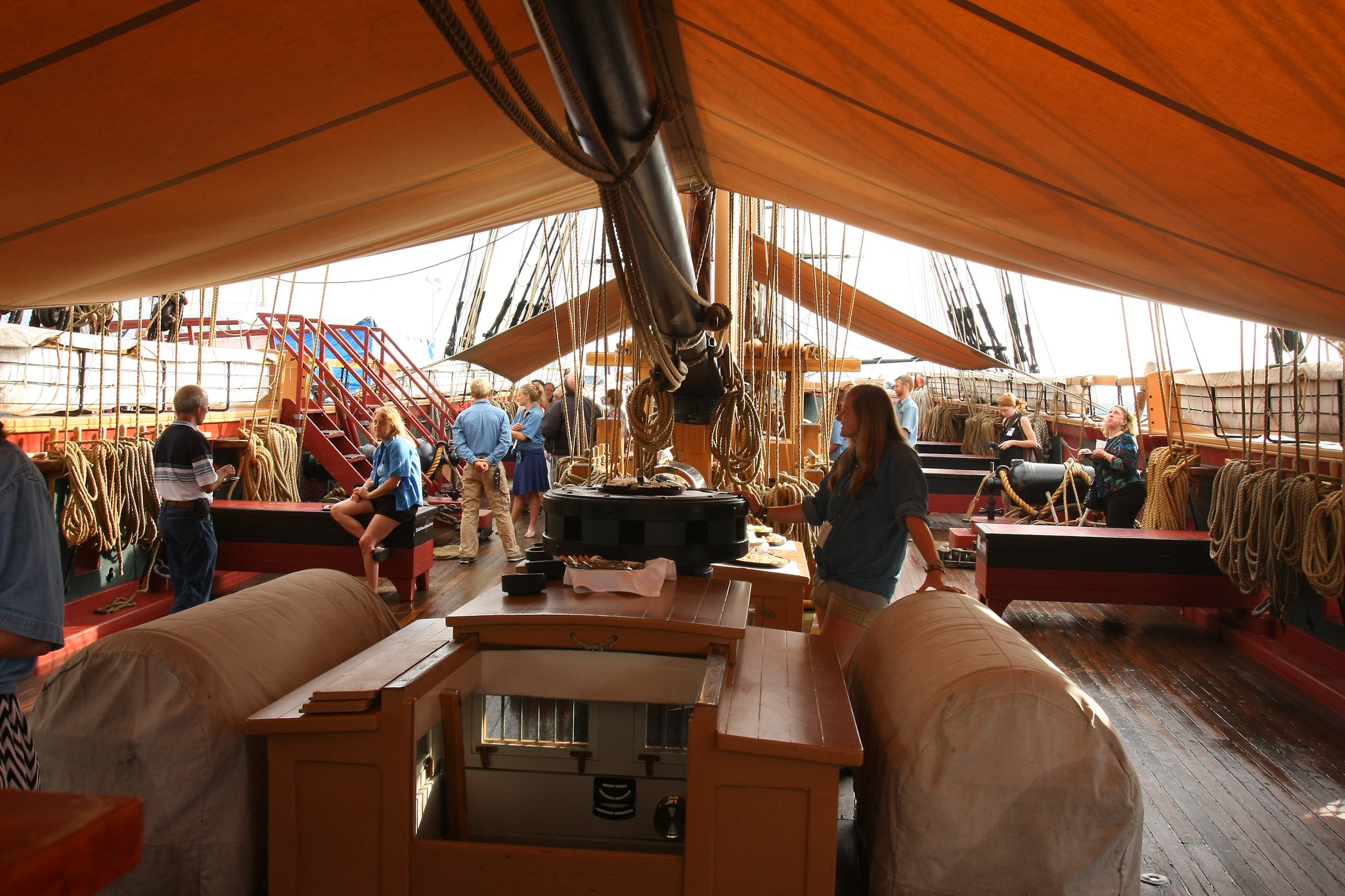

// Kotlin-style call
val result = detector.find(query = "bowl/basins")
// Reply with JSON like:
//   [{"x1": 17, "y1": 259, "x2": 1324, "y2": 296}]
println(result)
[
  {"x1": 754, "y1": 531, "x2": 770, "y2": 538},
  {"x1": 766, "y1": 539, "x2": 787, "y2": 546}
]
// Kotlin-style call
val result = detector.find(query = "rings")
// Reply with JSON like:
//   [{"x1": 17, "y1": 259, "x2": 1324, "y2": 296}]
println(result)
[{"x1": 352, "y1": 495, "x2": 355, "y2": 496}]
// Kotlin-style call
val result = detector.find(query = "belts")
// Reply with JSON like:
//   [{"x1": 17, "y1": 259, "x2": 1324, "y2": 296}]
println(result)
[{"x1": 162, "y1": 499, "x2": 195, "y2": 508}]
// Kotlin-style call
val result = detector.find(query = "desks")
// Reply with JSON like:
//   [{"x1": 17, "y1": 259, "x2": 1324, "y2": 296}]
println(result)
[
  {"x1": 974, "y1": 522, "x2": 1270, "y2": 618},
  {"x1": 917, "y1": 453, "x2": 1001, "y2": 471},
  {"x1": 914, "y1": 440, "x2": 964, "y2": 455},
  {"x1": 209, "y1": 498, "x2": 439, "y2": 601},
  {"x1": 921, "y1": 467, "x2": 1003, "y2": 515}
]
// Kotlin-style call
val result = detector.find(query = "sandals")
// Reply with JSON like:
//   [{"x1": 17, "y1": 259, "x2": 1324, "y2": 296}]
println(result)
[
  {"x1": 494, "y1": 530, "x2": 516, "y2": 534},
  {"x1": 525, "y1": 529, "x2": 538, "y2": 537}
]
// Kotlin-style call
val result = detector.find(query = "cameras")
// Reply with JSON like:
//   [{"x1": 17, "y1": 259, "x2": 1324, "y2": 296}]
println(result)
[{"x1": 985, "y1": 441, "x2": 1002, "y2": 458}]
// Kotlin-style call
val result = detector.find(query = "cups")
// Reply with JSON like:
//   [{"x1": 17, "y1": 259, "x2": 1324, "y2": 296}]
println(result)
[{"x1": 1095, "y1": 439, "x2": 1107, "y2": 450}]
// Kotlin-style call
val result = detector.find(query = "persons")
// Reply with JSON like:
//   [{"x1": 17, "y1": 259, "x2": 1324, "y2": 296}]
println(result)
[
  {"x1": 331, "y1": 406, "x2": 423, "y2": 593},
  {"x1": 892, "y1": 375, "x2": 919, "y2": 450},
  {"x1": 152, "y1": 385, "x2": 236, "y2": 616},
  {"x1": 452, "y1": 374, "x2": 622, "y2": 564},
  {"x1": 452, "y1": 378, "x2": 525, "y2": 564},
  {"x1": 991, "y1": 393, "x2": 1037, "y2": 518},
  {"x1": 0, "y1": 419, "x2": 64, "y2": 792},
  {"x1": 730, "y1": 370, "x2": 1140, "y2": 668}
]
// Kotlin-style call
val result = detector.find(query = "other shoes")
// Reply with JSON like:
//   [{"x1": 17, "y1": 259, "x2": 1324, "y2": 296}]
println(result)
[
  {"x1": 460, "y1": 557, "x2": 476, "y2": 563},
  {"x1": 373, "y1": 545, "x2": 393, "y2": 561},
  {"x1": 507, "y1": 552, "x2": 525, "y2": 561}
]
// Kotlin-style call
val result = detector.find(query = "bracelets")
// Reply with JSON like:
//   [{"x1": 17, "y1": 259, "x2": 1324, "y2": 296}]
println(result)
[
  {"x1": 752, "y1": 504, "x2": 769, "y2": 524},
  {"x1": 923, "y1": 559, "x2": 946, "y2": 574}
]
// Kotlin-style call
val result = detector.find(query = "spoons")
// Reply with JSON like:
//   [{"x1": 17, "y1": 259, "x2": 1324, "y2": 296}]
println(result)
[{"x1": 559, "y1": 554, "x2": 645, "y2": 570}]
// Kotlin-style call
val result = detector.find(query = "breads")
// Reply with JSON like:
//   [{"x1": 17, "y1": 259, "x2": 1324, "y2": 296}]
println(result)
[
  {"x1": 560, "y1": 554, "x2": 593, "y2": 564},
  {"x1": 1079, "y1": 448, "x2": 1092, "y2": 453}
]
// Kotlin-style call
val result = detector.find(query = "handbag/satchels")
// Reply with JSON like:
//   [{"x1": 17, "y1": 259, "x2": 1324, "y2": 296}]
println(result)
[
  {"x1": 1084, "y1": 475, "x2": 1104, "y2": 512},
  {"x1": 501, "y1": 446, "x2": 517, "y2": 462},
  {"x1": 1019, "y1": 416, "x2": 1041, "y2": 463}
]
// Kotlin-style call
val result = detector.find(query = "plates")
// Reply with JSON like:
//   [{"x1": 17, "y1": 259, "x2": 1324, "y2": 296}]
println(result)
[
  {"x1": 222, "y1": 477, "x2": 240, "y2": 483},
  {"x1": 1080, "y1": 453, "x2": 1093, "y2": 455},
  {"x1": 738, "y1": 554, "x2": 790, "y2": 568},
  {"x1": 321, "y1": 505, "x2": 332, "y2": 510}
]
